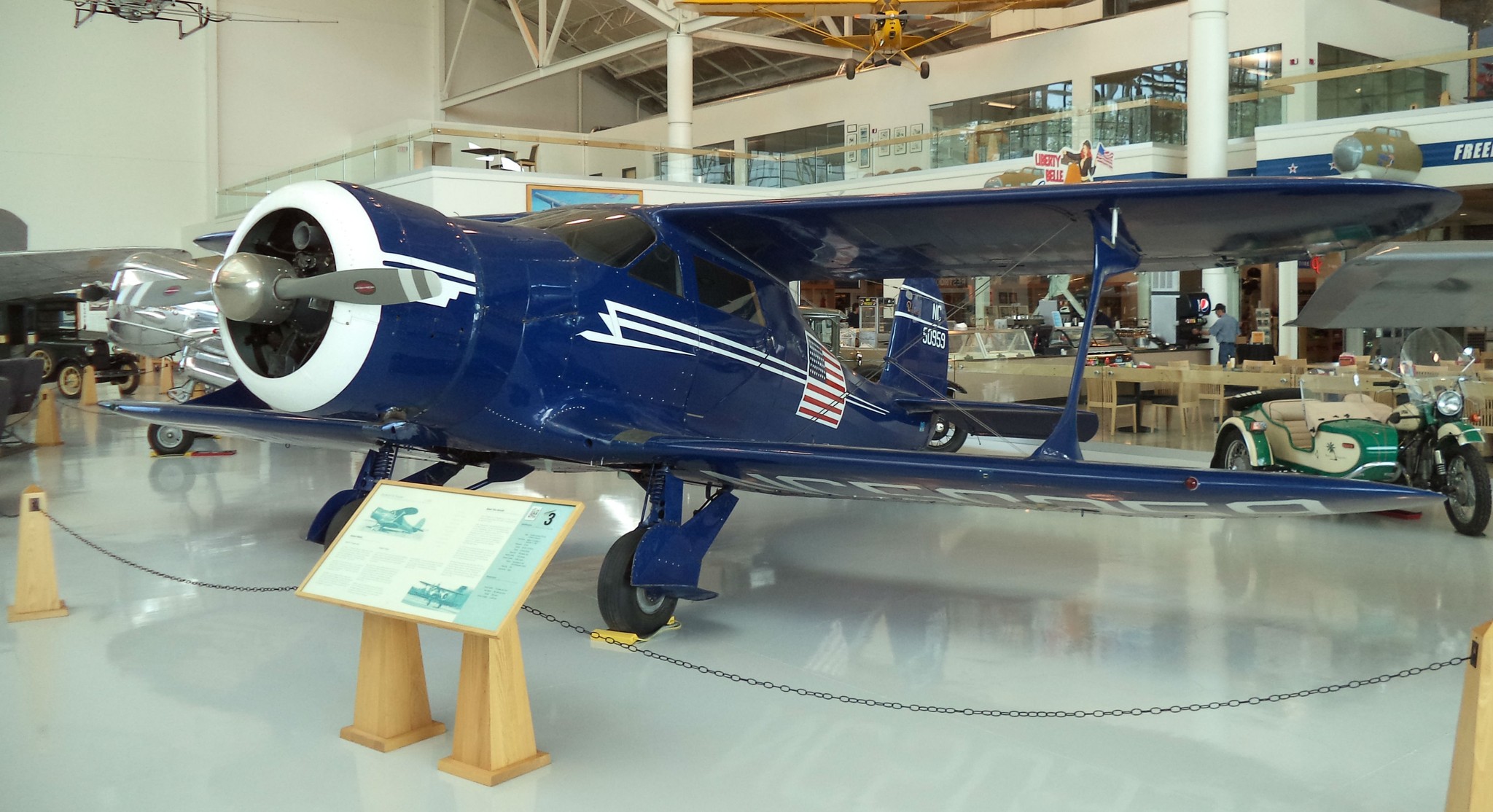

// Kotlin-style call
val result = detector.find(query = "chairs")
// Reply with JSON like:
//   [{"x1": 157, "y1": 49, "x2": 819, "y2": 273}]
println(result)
[
  {"x1": 1087, "y1": 365, "x2": 1137, "y2": 435},
  {"x1": 1150, "y1": 347, "x2": 1493, "y2": 458}
]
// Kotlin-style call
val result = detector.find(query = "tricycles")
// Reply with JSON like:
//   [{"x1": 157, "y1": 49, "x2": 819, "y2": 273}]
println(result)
[{"x1": 1204, "y1": 323, "x2": 1492, "y2": 536}]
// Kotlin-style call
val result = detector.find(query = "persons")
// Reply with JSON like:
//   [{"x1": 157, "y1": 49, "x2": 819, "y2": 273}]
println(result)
[
  {"x1": 837, "y1": 297, "x2": 849, "y2": 314},
  {"x1": 1191, "y1": 303, "x2": 1242, "y2": 396},
  {"x1": 1095, "y1": 305, "x2": 1112, "y2": 329},
  {"x1": 846, "y1": 303, "x2": 863, "y2": 347}
]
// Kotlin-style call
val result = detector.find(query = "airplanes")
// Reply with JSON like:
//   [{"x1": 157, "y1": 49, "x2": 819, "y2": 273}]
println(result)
[
  {"x1": 0, "y1": 178, "x2": 1467, "y2": 640},
  {"x1": 674, "y1": 0, "x2": 1074, "y2": 82}
]
